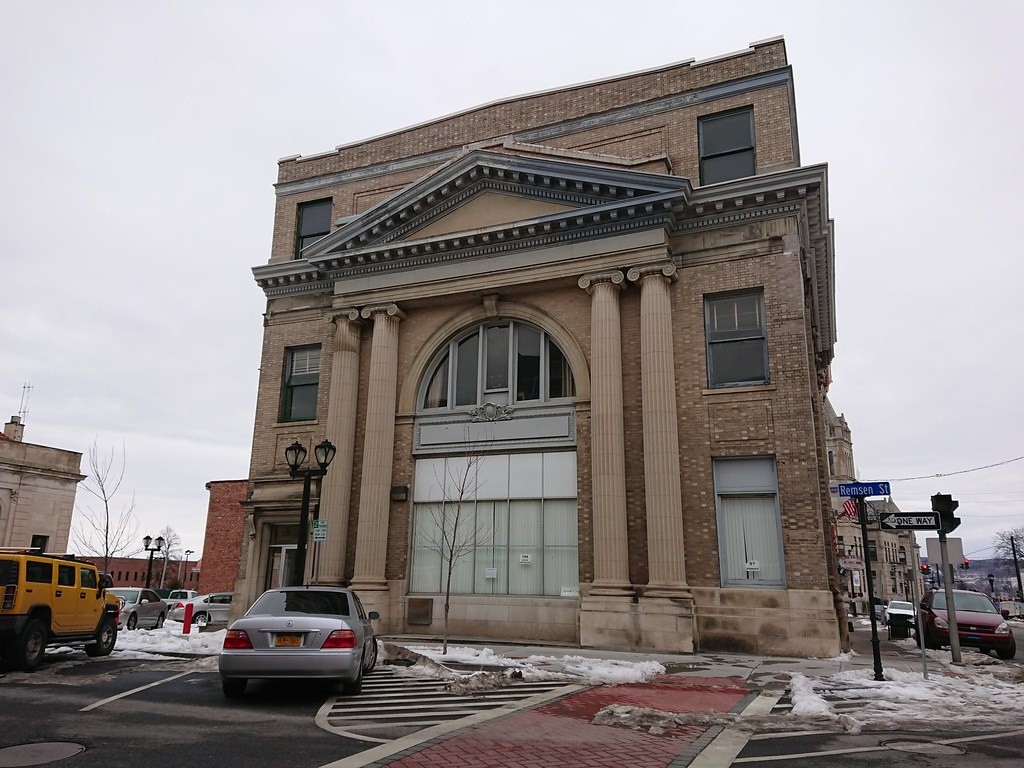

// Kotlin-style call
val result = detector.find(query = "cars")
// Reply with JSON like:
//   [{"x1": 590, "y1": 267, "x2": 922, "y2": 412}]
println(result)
[
  {"x1": 218, "y1": 587, "x2": 380, "y2": 697},
  {"x1": 874, "y1": 595, "x2": 883, "y2": 619},
  {"x1": 104, "y1": 586, "x2": 168, "y2": 631},
  {"x1": 916, "y1": 586, "x2": 1016, "y2": 660},
  {"x1": 883, "y1": 600, "x2": 917, "y2": 626},
  {"x1": 166, "y1": 592, "x2": 233, "y2": 624}
]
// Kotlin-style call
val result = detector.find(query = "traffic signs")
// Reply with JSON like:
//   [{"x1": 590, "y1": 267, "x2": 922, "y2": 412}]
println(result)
[{"x1": 879, "y1": 511, "x2": 942, "y2": 531}]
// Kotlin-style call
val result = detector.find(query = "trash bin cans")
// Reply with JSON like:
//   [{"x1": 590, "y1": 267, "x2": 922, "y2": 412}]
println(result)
[{"x1": 888, "y1": 612, "x2": 913, "y2": 641}]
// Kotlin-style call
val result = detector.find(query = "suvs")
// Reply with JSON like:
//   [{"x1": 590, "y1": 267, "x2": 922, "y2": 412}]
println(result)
[
  {"x1": 97, "y1": 571, "x2": 113, "y2": 589},
  {"x1": 0, "y1": 546, "x2": 126, "y2": 672}
]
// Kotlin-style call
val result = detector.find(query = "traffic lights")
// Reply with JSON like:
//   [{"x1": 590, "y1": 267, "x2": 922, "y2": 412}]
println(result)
[
  {"x1": 959, "y1": 557, "x2": 969, "y2": 571},
  {"x1": 920, "y1": 564, "x2": 931, "y2": 575},
  {"x1": 941, "y1": 493, "x2": 961, "y2": 534}
]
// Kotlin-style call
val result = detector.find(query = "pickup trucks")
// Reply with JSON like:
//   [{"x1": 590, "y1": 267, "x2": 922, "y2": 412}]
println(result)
[{"x1": 161, "y1": 590, "x2": 198, "y2": 611}]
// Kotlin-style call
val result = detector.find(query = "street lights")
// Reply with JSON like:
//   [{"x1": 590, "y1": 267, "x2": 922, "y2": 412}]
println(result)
[
  {"x1": 182, "y1": 550, "x2": 195, "y2": 589},
  {"x1": 142, "y1": 535, "x2": 166, "y2": 589},
  {"x1": 285, "y1": 438, "x2": 337, "y2": 588},
  {"x1": 987, "y1": 573, "x2": 994, "y2": 592}
]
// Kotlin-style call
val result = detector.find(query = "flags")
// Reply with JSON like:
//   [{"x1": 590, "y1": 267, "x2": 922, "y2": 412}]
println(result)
[{"x1": 843, "y1": 495, "x2": 860, "y2": 519}]
